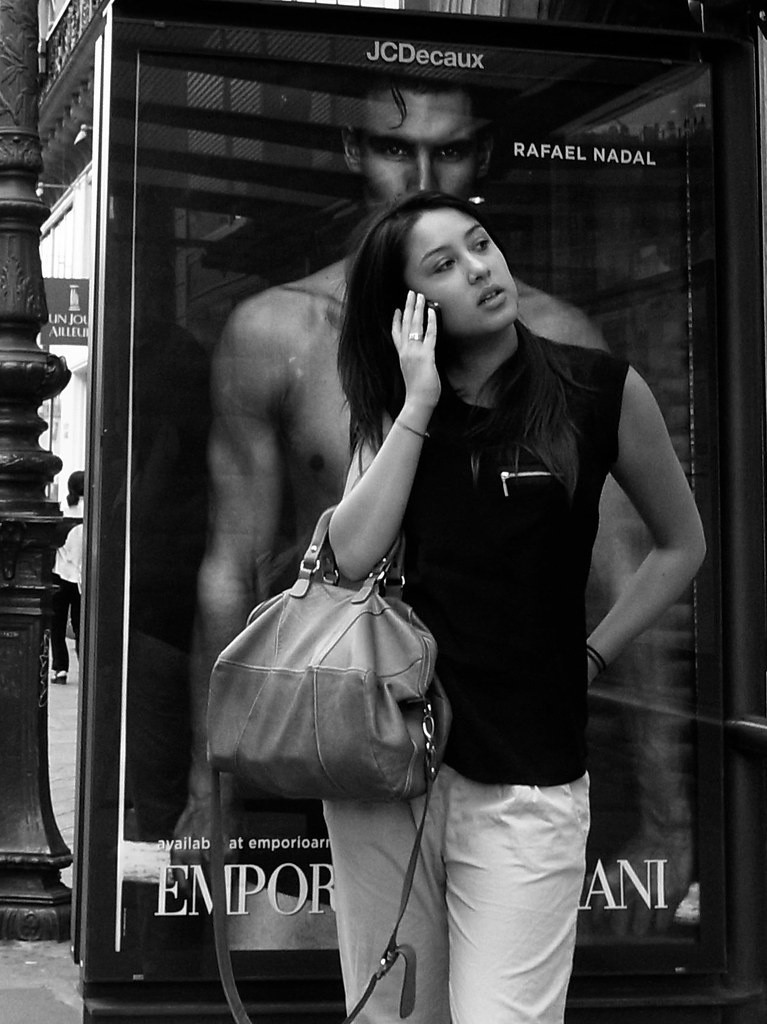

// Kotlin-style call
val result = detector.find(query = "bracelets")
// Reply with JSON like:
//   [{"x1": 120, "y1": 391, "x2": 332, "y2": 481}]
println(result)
[
  {"x1": 584, "y1": 643, "x2": 607, "y2": 676},
  {"x1": 585, "y1": 651, "x2": 604, "y2": 677},
  {"x1": 392, "y1": 418, "x2": 429, "y2": 439}
]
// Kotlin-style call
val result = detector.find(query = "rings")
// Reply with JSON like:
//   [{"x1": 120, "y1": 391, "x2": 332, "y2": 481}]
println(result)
[{"x1": 408, "y1": 331, "x2": 425, "y2": 342}]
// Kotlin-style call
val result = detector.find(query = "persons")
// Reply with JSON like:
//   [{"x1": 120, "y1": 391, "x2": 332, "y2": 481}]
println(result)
[
  {"x1": 47, "y1": 472, "x2": 84, "y2": 685},
  {"x1": 319, "y1": 187, "x2": 708, "y2": 1024},
  {"x1": 168, "y1": 74, "x2": 694, "y2": 957}
]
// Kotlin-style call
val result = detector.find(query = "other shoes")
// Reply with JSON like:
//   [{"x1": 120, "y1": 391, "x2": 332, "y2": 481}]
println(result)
[{"x1": 51, "y1": 670, "x2": 67, "y2": 685}]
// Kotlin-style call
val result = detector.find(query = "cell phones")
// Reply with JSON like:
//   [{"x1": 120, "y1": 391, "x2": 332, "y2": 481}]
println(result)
[{"x1": 401, "y1": 293, "x2": 443, "y2": 338}]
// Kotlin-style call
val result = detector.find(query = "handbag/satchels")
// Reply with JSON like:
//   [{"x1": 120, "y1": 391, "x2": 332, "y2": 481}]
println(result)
[{"x1": 206, "y1": 509, "x2": 450, "y2": 803}]
